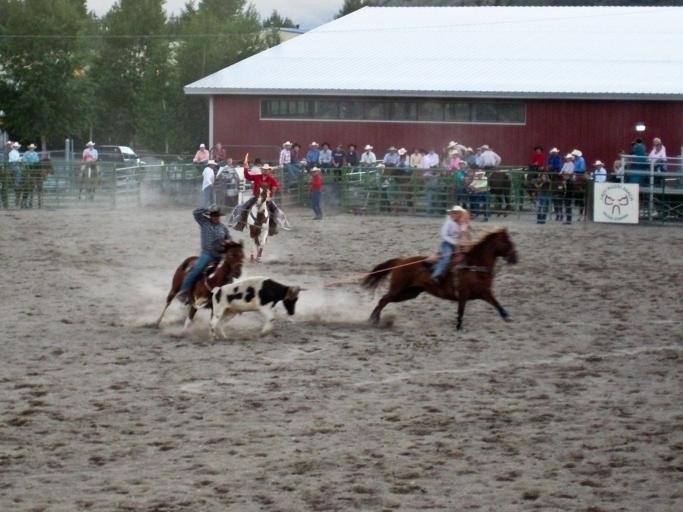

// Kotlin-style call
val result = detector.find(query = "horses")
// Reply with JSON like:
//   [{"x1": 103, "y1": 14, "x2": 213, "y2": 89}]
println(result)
[
  {"x1": 77, "y1": 162, "x2": 101, "y2": 202},
  {"x1": 376, "y1": 163, "x2": 513, "y2": 218},
  {"x1": 523, "y1": 162, "x2": 590, "y2": 224},
  {"x1": 0, "y1": 157, "x2": 56, "y2": 209},
  {"x1": 239, "y1": 185, "x2": 271, "y2": 264},
  {"x1": 361, "y1": 226, "x2": 520, "y2": 330},
  {"x1": 154, "y1": 238, "x2": 245, "y2": 330}
]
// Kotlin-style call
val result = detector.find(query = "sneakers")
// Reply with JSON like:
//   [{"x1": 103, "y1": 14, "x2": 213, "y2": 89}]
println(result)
[
  {"x1": 483, "y1": 208, "x2": 585, "y2": 224},
  {"x1": 179, "y1": 291, "x2": 187, "y2": 303},
  {"x1": 314, "y1": 214, "x2": 324, "y2": 221}
]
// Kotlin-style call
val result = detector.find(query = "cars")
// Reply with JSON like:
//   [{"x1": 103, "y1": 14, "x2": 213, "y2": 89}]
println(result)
[{"x1": 17, "y1": 142, "x2": 199, "y2": 193}]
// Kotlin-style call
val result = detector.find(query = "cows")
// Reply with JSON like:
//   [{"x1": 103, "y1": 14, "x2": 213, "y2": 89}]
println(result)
[{"x1": 193, "y1": 277, "x2": 308, "y2": 344}]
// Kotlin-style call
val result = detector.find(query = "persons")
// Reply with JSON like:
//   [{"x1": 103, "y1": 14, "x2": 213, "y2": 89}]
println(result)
[
  {"x1": 192, "y1": 137, "x2": 261, "y2": 223},
  {"x1": 7, "y1": 141, "x2": 22, "y2": 187},
  {"x1": 527, "y1": 137, "x2": 669, "y2": 226},
  {"x1": 179, "y1": 203, "x2": 234, "y2": 291},
  {"x1": 429, "y1": 204, "x2": 467, "y2": 281},
  {"x1": 3, "y1": 141, "x2": 13, "y2": 162},
  {"x1": 280, "y1": 141, "x2": 502, "y2": 223},
  {"x1": 233, "y1": 152, "x2": 281, "y2": 236},
  {"x1": 77, "y1": 139, "x2": 99, "y2": 179},
  {"x1": 23, "y1": 143, "x2": 40, "y2": 163}
]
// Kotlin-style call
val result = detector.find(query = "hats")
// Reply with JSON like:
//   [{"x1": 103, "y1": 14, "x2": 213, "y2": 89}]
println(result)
[
  {"x1": 85, "y1": 140, "x2": 96, "y2": 148},
  {"x1": 346, "y1": 144, "x2": 356, "y2": 148},
  {"x1": 293, "y1": 143, "x2": 301, "y2": 149},
  {"x1": 322, "y1": 143, "x2": 331, "y2": 148},
  {"x1": 206, "y1": 160, "x2": 218, "y2": 166},
  {"x1": 205, "y1": 204, "x2": 225, "y2": 216},
  {"x1": 3, "y1": 140, "x2": 37, "y2": 151},
  {"x1": 535, "y1": 145, "x2": 583, "y2": 159},
  {"x1": 283, "y1": 141, "x2": 293, "y2": 147},
  {"x1": 446, "y1": 204, "x2": 467, "y2": 213},
  {"x1": 592, "y1": 160, "x2": 607, "y2": 167},
  {"x1": 200, "y1": 143, "x2": 205, "y2": 148},
  {"x1": 259, "y1": 163, "x2": 272, "y2": 170},
  {"x1": 364, "y1": 144, "x2": 408, "y2": 156},
  {"x1": 447, "y1": 141, "x2": 492, "y2": 176},
  {"x1": 311, "y1": 165, "x2": 321, "y2": 172},
  {"x1": 309, "y1": 141, "x2": 319, "y2": 147}
]
